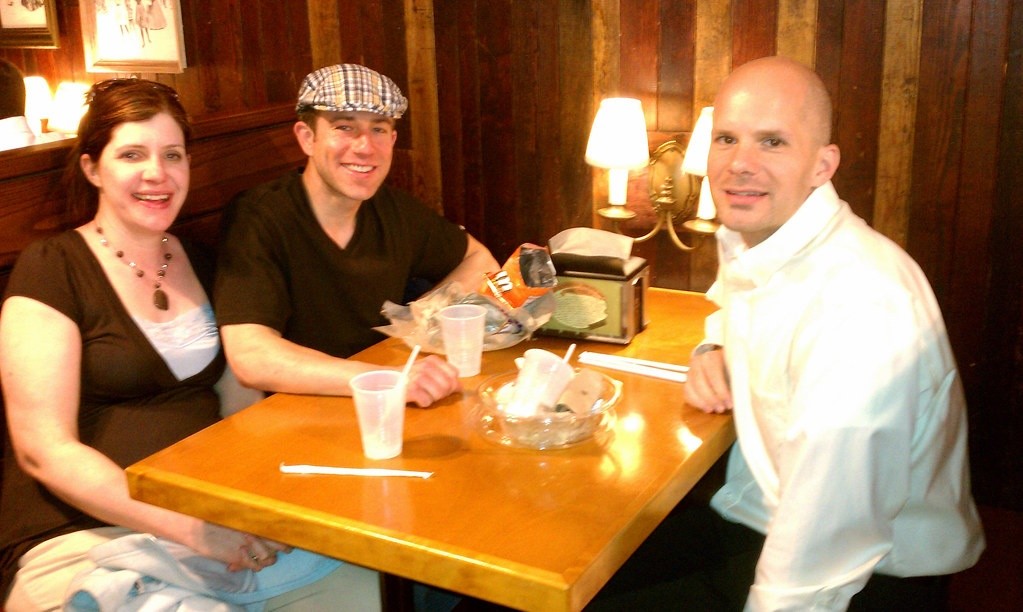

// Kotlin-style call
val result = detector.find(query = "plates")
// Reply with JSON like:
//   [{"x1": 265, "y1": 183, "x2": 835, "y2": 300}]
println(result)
[{"x1": 466, "y1": 401, "x2": 618, "y2": 454}]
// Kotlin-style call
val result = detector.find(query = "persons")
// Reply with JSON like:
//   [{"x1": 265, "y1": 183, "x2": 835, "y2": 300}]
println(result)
[
  {"x1": 1, "y1": 61, "x2": 37, "y2": 152},
  {"x1": 224, "y1": 64, "x2": 512, "y2": 409},
  {"x1": 582, "y1": 55, "x2": 991, "y2": 612},
  {"x1": 0, "y1": 77, "x2": 383, "y2": 612},
  {"x1": 118, "y1": 0, "x2": 165, "y2": 47}
]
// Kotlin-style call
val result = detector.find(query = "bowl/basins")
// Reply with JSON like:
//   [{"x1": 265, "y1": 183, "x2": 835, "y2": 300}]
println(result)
[{"x1": 475, "y1": 368, "x2": 624, "y2": 446}]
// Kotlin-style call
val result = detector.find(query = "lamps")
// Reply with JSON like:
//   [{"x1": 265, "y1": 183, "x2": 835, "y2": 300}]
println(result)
[{"x1": 584, "y1": 94, "x2": 719, "y2": 253}]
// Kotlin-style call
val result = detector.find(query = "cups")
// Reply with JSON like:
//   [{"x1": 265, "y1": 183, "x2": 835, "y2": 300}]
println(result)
[
  {"x1": 438, "y1": 304, "x2": 488, "y2": 378},
  {"x1": 505, "y1": 349, "x2": 576, "y2": 417},
  {"x1": 350, "y1": 370, "x2": 409, "y2": 460}
]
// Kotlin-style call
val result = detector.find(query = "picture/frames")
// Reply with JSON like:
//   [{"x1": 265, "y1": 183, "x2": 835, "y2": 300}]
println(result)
[
  {"x1": 82, "y1": 0, "x2": 186, "y2": 74},
  {"x1": 0, "y1": 0, "x2": 59, "y2": 50}
]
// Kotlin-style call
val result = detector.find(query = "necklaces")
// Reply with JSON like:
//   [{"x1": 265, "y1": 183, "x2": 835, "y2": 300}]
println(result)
[{"x1": 94, "y1": 219, "x2": 174, "y2": 309}]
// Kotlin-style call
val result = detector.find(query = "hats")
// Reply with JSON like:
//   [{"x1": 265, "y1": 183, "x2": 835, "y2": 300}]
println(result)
[{"x1": 295, "y1": 63, "x2": 408, "y2": 119}]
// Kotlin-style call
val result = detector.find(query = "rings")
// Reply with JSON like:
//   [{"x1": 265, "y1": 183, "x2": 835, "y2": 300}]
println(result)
[{"x1": 252, "y1": 556, "x2": 258, "y2": 561}]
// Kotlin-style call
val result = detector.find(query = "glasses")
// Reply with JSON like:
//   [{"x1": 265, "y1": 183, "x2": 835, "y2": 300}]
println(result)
[{"x1": 90, "y1": 78, "x2": 178, "y2": 104}]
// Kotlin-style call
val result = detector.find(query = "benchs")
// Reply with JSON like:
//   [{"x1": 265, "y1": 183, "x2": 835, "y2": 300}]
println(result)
[{"x1": 0, "y1": 105, "x2": 307, "y2": 255}]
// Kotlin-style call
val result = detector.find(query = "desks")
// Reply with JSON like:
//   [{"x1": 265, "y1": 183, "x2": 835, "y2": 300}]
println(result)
[{"x1": 125, "y1": 276, "x2": 735, "y2": 612}]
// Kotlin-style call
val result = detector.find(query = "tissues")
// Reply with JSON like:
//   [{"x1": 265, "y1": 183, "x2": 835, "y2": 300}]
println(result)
[{"x1": 532, "y1": 227, "x2": 650, "y2": 345}]
